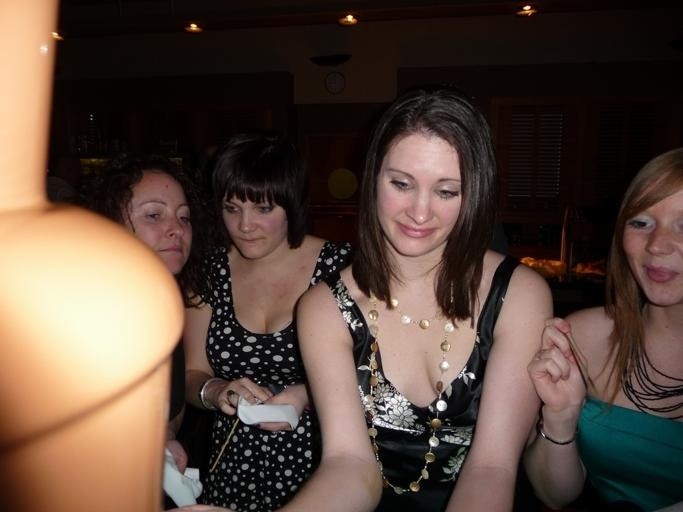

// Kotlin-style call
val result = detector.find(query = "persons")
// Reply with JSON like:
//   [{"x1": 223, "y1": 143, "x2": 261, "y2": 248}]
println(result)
[{"x1": 94, "y1": 90, "x2": 683, "y2": 512}]
